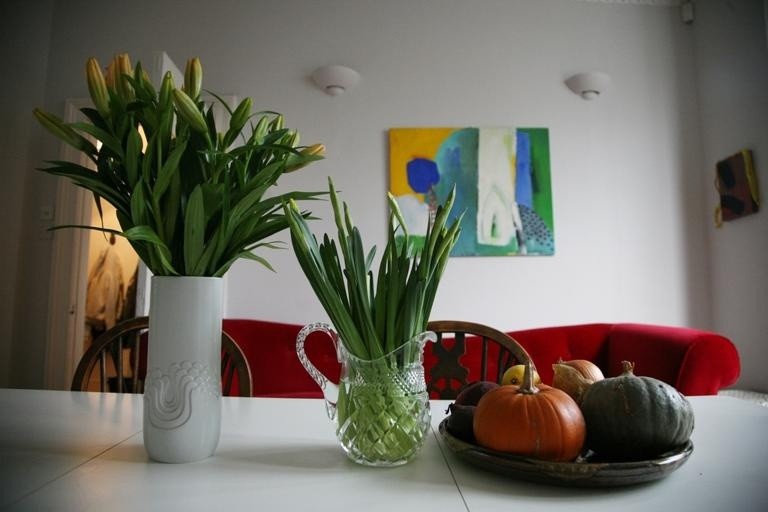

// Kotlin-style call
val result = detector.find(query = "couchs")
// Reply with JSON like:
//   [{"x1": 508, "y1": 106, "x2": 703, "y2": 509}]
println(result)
[{"x1": 129, "y1": 318, "x2": 741, "y2": 397}]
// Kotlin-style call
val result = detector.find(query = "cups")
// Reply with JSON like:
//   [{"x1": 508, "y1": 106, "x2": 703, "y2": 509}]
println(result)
[{"x1": 294, "y1": 323, "x2": 438, "y2": 468}]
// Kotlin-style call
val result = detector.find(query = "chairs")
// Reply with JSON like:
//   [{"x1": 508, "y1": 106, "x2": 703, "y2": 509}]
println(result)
[
  {"x1": 426, "y1": 320, "x2": 536, "y2": 399},
  {"x1": 73, "y1": 316, "x2": 255, "y2": 397}
]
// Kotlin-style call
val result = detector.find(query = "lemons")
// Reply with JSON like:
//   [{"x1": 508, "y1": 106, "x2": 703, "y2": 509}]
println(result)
[{"x1": 502, "y1": 364, "x2": 541, "y2": 386}]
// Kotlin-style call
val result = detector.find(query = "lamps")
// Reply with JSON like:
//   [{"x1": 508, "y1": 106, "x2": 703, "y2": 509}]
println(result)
[
  {"x1": 315, "y1": 66, "x2": 359, "y2": 96},
  {"x1": 564, "y1": 72, "x2": 611, "y2": 99}
]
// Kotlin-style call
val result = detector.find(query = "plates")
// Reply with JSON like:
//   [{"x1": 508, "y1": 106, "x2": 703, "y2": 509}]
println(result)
[{"x1": 438, "y1": 417, "x2": 694, "y2": 488}]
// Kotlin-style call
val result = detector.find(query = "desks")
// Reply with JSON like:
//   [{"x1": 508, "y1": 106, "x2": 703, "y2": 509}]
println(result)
[{"x1": 0, "y1": 387, "x2": 767, "y2": 511}]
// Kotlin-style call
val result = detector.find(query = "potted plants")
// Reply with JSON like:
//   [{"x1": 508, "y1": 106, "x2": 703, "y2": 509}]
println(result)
[
  {"x1": 282, "y1": 178, "x2": 469, "y2": 471},
  {"x1": 32, "y1": 51, "x2": 346, "y2": 463}
]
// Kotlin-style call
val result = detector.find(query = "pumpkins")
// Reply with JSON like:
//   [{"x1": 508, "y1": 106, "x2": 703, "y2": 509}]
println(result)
[
  {"x1": 553, "y1": 357, "x2": 604, "y2": 407},
  {"x1": 473, "y1": 358, "x2": 588, "y2": 463},
  {"x1": 580, "y1": 359, "x2": 696, "y2": 461}
]
